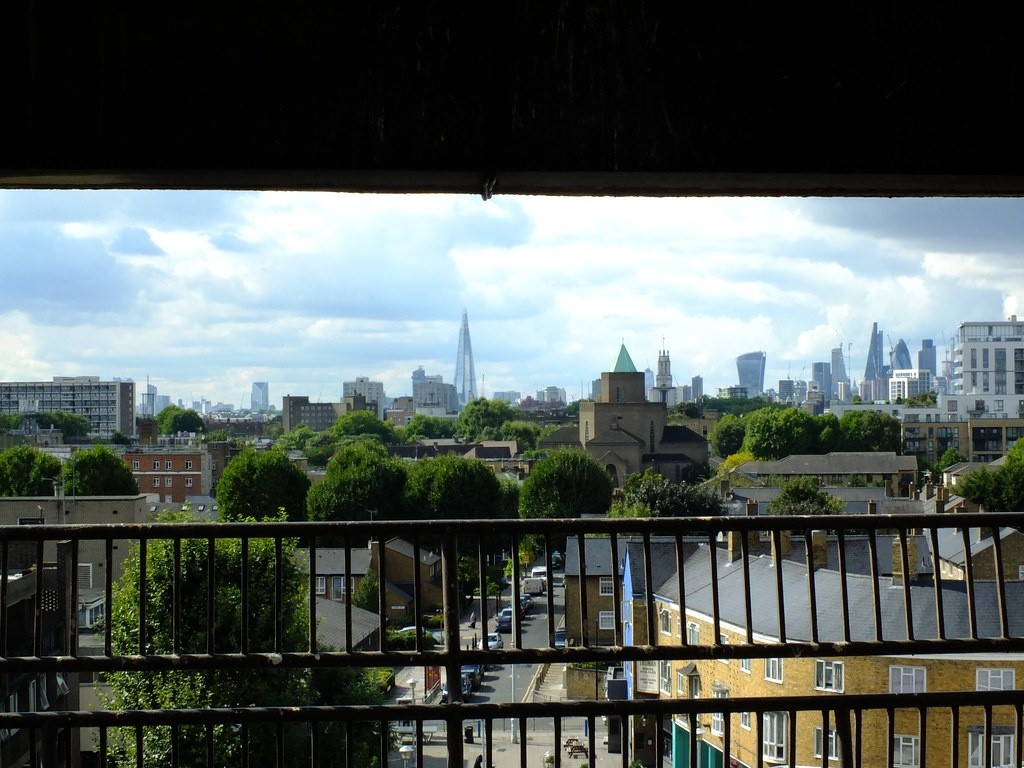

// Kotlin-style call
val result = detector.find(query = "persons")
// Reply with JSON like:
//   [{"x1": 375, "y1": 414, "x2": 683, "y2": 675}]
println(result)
[{"x1": 470, "y1": 611, "x2": 477, "y2": 629}]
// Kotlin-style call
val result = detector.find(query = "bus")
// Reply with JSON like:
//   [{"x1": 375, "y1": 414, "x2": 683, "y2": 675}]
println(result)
[{"x1": 531, "y1": 566, "x2": 547, "y2": 591}]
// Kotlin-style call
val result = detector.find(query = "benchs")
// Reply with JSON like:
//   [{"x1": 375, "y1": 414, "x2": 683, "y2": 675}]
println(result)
[
  {"x1": 567, "y1": 747, "x2": 572, "y2": 758},
  {"x1": 563, "y1": 741, "x2": 568, "y2": 751}
]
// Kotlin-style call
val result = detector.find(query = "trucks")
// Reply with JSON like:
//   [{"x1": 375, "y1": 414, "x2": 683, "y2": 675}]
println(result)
[{"x1": 524, "y1": 578, "x2": 544, "y2": 596}]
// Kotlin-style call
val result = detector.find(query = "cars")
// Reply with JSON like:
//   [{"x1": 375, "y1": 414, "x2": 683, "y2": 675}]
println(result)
[
  {"x1": 562, "y1": 577, "x2": 565, "y2": 587},
  {"x1": 508, "y1": 605, "x2": 525, "y2": 620},
  {"x1": 495, "y1": 616, "x2": 512, "y2": 631},
  {"x1": 520, "y1": 594, "x2": 535, "y2": 609},
  {"x1": 461, "y1": 665, "x2": 485, "y2": 682},
  {"x1": 441, "y1": 673, "x2": 472, "y2": 702},
  {"x1": 478, "y1": 633, "x2": 504, "y2": 649},
  {"x1": 501, "y1": 608, "x2": 512, "y2": 620},
  {"x1": 552, "y1": 553, "x2": 560, "y2": 558},
  {"x1": 460, "y1": 670, "x2": 481, "y2": 692},
  {"x1": 520, "y1": 597, "x2": 529, "y2": 613}
]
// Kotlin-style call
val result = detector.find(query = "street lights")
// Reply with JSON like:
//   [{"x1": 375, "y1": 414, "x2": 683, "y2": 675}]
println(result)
[
  {"x1": 407, "y1": 678, "x2": 419, "y2": 768},
  {"x1": 848, "y1": 342, "x2": 852, "y2": 399},
  {"x1": 398, "y1": 745, "x2": 414, "y2": 768},
  {"x1": 508, "y1": 641, "x2": 518, "y2": 745}
]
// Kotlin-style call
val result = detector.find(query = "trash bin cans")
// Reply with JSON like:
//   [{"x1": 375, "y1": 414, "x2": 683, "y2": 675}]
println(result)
[{"x1": 464, "y1": 725, "x2": 474, "y2": 744}]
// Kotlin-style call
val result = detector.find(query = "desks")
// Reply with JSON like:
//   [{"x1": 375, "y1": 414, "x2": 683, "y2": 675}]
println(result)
[
  {"x1": 565, "y1": 735, "x2": 579, "y2": 751},
  {"x1": 569, "y1": 741, "x2": 588, "y2": 758},
  {"x1": 394, "y1": 726, "x2": 438, "y2": 744}
]
